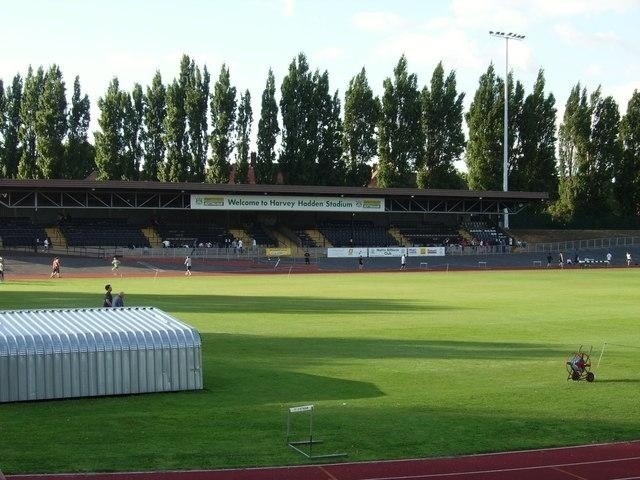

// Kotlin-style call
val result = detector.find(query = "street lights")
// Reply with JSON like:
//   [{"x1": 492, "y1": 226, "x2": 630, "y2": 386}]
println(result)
[{"x1": 489, "y1": 29, "x2": 525, "y2": 229}]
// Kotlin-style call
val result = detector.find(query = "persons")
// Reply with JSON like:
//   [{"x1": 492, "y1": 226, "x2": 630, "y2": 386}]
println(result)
[
  {"x1": 304, "y1": 250, "x2": 310, "y2": 264},
  {"x1": 184, "y1": 256, "x2": 192, "y2": 276},
  {"x1": 400, "y1": 254, "x2": 407, "y2": 271},
  {"x1": 112, "y1": 291, "x2": 124, "y2": 307},
  {"x1": 0, "y1": 258, "x2": 5, "y2": 280},
  {"x1": 104, "y1": 285, "x2": 113, "y2": 307},
  {"x1": 111, "y1": 256, "x2": 122, "y2": 278},
  {"x1": 41, "y1": 237, "x2": 50, "y2": 255},
  {"x1": 626, "y1": 250, "x2": 632, "y2": 267},
  {"x1": 559, "y1": 251, "x2": 565, "y2": 269},
  {"x1": 606, "y1": 251, "x2": 613, "y2": 267},
  {"x1": 546, "y1": 253, "x2": 553, "y2": 269},
  {"x1": 49, "y1": 257, "x2": 60, "y2": 279},
  {"x1": 359, "y1": 255, "x2": 364, "y2": 272},
  {"x1": 162, "y1": 236, "x2": 257, "y2": 255},
  {"x1": 442, "y1": 234, "x2": 529, "y2": 248}
]
